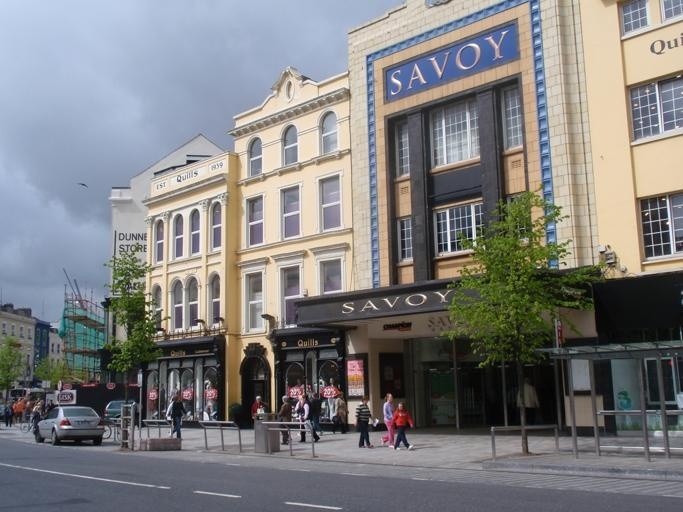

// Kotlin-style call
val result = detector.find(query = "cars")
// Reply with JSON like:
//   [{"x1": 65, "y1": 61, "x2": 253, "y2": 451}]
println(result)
[{"x1": 33, "y1": 405, "x2": 106, "y2": 446}]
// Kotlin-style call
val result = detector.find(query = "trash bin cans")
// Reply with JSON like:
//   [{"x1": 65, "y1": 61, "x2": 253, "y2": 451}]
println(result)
[{"x1": 253, "y1": 413, "x2": 281, "y2": 453}]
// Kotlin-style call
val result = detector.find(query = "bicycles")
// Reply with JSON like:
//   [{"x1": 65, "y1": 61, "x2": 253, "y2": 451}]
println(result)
[
  {"x1": 103, "y1": 418, "x2": 112, "y2": 440},
  {"x1": 20, "y1": 412, "x2": 34, "y2": 434}
]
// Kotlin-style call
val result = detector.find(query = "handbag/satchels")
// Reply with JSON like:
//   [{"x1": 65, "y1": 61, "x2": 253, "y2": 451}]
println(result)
[{"x1": 333, "y1": 416, "x2": 340, "y2": 422}]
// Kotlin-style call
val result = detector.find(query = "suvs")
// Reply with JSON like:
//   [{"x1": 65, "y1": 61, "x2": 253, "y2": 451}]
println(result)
[{"x1": 104, "y1": 399, "x2": 139, "y2": 427}]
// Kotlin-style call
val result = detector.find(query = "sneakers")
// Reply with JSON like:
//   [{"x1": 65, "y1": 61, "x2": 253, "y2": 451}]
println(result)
[
  {"x1": 389, "y1": 445, "x2": 400, "y2": 450},
  {"x1": 359, "y1": 445, "x2": 364, "y2": 448},
  {"x1": 381, "y1": 436, "x2": 384, "y2": 446},
  {"x1": 408, "y1": 446, "x2": 413, "y2": 451},
  {"x1": 368, "y1": 445, "x2": 373, "y2": 448},
  {"x1": 300, "y1": 440, "x2": 304, "y2": 442},
  {"x1": 315, "y1": 437, "x2": 319, "y2": 442}
]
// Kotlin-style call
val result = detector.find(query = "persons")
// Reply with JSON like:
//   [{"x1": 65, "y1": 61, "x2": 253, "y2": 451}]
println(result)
[
  {"x1": 516, "y1": 377, "x2": 540, "y2": 426},
  {"x1": 171, "y1": 396, "x2": 187, "y2": 441},
  {"x1": 251, "y1": 396, "x2": 268, "y2": 419},
  {"x1": 295, "y1": 379, "x2": 304, "y2": 390},
  {"x1": 4, "y1": 398, "x2": 54, "y2": 429},
  {"x1": 148, "y1": 383, "x2": 217, "y2": 420},
  {"x1": 327, "y1": 378, "x2": 337, "y2": 421},
  {"x1": 166, "y1": 396, "x2": 182, "y2": 433},
  {"x1": 278, "y1": 393, "x2": 415, "y2": 451}
]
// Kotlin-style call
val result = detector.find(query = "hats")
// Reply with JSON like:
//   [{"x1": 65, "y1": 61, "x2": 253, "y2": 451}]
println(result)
[{"x1": 256, "y1": 396, "x2": 261, "y2": 400}]
